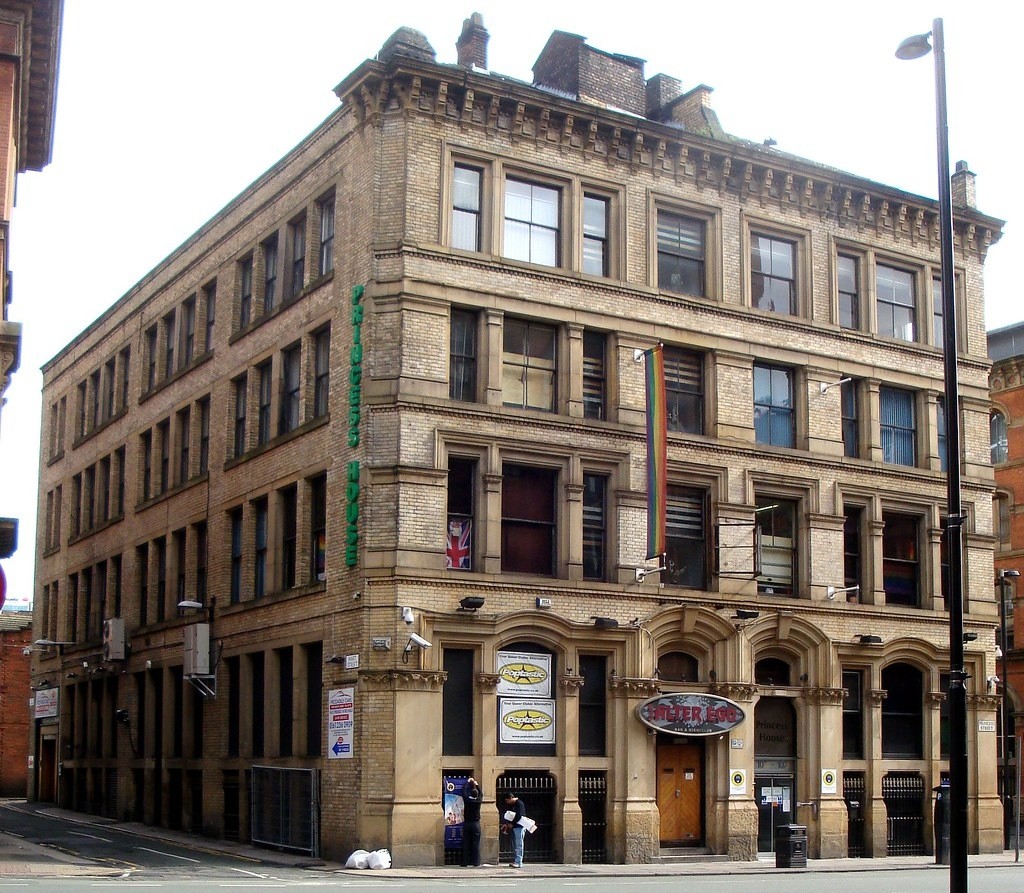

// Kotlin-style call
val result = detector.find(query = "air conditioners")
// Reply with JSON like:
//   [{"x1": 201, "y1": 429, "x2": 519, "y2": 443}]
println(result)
[{"x1": 102, "y1": 618, "x2": 125, "y2": 660}]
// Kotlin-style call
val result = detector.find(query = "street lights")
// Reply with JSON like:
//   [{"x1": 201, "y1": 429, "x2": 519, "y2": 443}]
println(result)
[
  {"x1": 894, "y1": 16, "x2": 975, "y2": 893},
  {"x1": 998, "y1": 568, "x2": 1022, "y2": 852}
]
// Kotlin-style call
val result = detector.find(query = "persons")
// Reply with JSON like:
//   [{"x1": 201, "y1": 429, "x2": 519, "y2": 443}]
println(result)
[
  {"x1": 503, "y1": 793, "x2": 526, "y2": 868},
  {"x1": 448, "y1": 804, "x2": 462, "y2": 825},
  {"x1": 460, "y1": 778, "x2": 483, "y2": 869}
]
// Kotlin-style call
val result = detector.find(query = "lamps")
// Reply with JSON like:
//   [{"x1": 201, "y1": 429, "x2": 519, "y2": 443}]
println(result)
[
  {"x1": 591, "y1": 616, "x2": 618, "y2": 628},
  {"x1": 177, "y1": 600, "x2": 210, "y2": 609},
  {"x1": 35, "y1": 639, "x2": 75, "y2": 646},
  {"x1": 456, "y1": 596, "x2": 484, "y2": 611},
  {"x1": 964, "y1": 632, "x2": 977, "y2": 643},
  {"x1": 855, "y1": 634, "x2": 883, "y2": 642},
  {"x1": 731, "y1": 609, "x2": 759, "y2": 619}
]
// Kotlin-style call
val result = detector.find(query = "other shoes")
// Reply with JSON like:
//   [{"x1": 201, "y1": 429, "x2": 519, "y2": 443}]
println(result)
[{"x1": 510, "y1": 862, "x2": 522, "y2": 868}]
